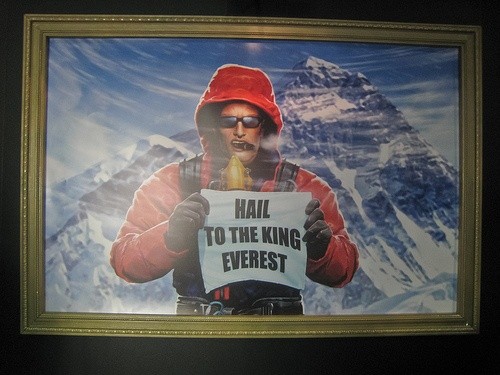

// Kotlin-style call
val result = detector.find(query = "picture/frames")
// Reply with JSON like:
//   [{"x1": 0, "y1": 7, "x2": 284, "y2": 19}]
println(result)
[{"x1": 21, "y1": 14, "x2": 483, "y2": 339}]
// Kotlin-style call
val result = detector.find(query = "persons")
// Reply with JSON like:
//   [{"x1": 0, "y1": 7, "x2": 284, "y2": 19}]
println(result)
[{"x1": 111, "y1": 63, "x2": 358, "y2": 315}]
